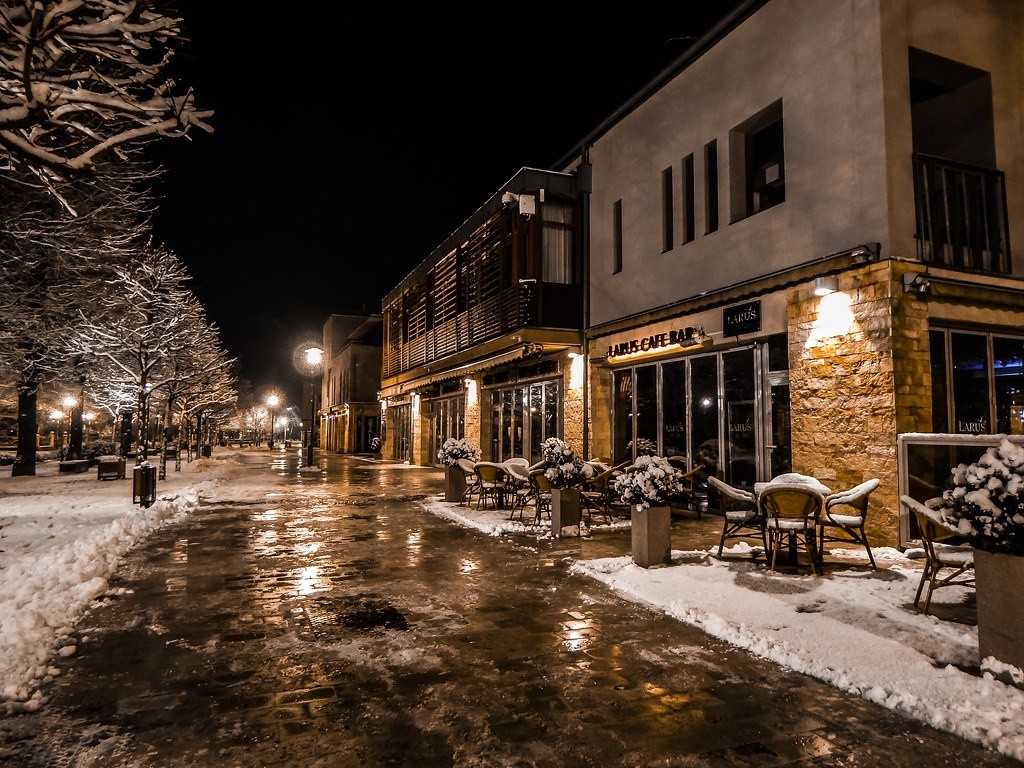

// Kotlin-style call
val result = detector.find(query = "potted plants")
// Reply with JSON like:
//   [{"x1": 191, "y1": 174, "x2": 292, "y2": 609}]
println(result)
[
  {"x1": 541, "y1": 438, "x2": 587, "y2": 537},
  {"x1": 940, "y1": 440, "x2": 1024, "y2": 692},
  {"x1": 696, "y1": 437, "x2": 738, "y2": 510},
  {"x1": 624, "y1": 437, "x2": 658, "y2": 456},
  {"x1": 614, "y1": 455, "x2": 683, "y2": 567},
  {"x1": 370, "y1": 436, "x2": 382, "y2": 461},
  {"x1": 438, "y1": 437, "x2": 482, "y2": 502}
]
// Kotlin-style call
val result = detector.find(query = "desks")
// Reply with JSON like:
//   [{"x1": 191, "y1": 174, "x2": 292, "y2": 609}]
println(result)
[{"x1": 755, "y1": 482, "x2": 831, "y2": 567}]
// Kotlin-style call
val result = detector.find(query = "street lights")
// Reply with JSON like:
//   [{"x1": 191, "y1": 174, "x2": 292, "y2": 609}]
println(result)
[
  {"x1": 62, "y1": 396, "x2": 77, "y2": 444},
  {"x1": 86, "y1": 412, "x2": 94, "y2": 446},
  {"x1": 267, "y1": 394, "x2": 278, "y2": 448},
  {"x1": 304, "y1": 347, "x2": 326, "y2": 465},
  {"x1": 49, "y1": 410, "x2": 63, "y2": 449}
]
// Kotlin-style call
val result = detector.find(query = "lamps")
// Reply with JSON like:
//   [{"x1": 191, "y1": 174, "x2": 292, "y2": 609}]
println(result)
[{"x1": 815, "y1": 277, "x2": 837, "y2": 295}]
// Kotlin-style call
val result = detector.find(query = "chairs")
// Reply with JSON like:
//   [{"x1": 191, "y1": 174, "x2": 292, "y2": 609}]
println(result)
[
  {"x1": 708, "y1": 473, "x2": 879, "y2": 574},
  {"x1": 901, "y1": 495, "x2": 976, "y2": 615},
  {"x1": 459, "y1": 457, "x2": 705, "y2": 532}
]
